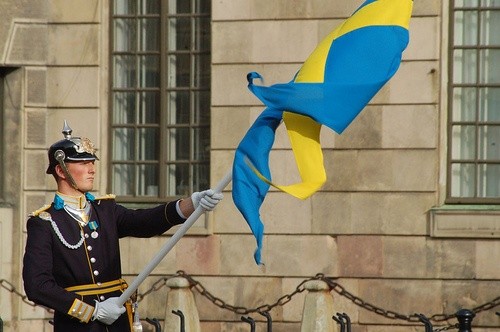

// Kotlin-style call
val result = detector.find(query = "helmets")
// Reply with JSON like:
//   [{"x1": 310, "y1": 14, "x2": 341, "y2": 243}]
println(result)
[{"x1": 46, "y1": 120, "x2": 100, "y2": 174}]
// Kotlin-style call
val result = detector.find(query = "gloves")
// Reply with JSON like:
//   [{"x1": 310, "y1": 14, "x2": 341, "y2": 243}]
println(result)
[
  {"x1": 191, "y1": 189, "x2": 224, "y2": 214},
  {"x1": 93, "y1": 297, "x2": 126, "y2": 325}
]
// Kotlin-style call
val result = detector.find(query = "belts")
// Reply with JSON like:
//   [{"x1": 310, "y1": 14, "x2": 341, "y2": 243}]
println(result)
[{"x1": 64, "y1": 278, "x2": 128, "y2": 296}]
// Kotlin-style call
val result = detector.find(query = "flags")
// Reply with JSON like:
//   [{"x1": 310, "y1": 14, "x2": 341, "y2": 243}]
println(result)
[{"x1": 232, "y1": 0, "x2": 414, "y2": 266}]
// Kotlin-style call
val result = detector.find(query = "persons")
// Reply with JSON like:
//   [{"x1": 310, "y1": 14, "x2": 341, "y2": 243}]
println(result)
[{"x1": 22, "y1": 137, "x2": 224, "y2": 332}]
